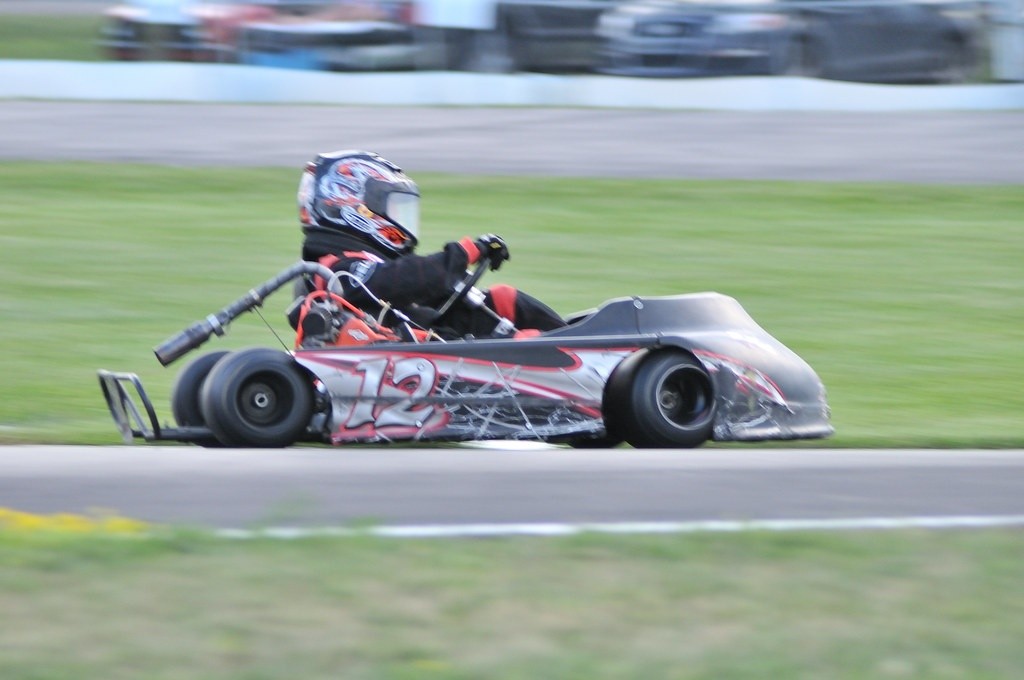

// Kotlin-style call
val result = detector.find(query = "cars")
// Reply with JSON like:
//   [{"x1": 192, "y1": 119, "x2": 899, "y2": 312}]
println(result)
[
  {"x1": 96, "y1": 1, "x2": 390, "y2": 61},
  {"x1": 596, "y1": 1, "x2": 979, "y2": 82}
]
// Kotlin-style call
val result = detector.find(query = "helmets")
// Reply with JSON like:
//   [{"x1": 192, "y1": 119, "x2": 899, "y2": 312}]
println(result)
[{"x1": 298, "y1": 149, "x2": 420, "y2": 259}]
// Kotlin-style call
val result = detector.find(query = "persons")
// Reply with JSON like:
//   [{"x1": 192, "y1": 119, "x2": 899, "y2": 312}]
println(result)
[{"x1": 297, "y1": 148, "x2": 572, "y2": 342}]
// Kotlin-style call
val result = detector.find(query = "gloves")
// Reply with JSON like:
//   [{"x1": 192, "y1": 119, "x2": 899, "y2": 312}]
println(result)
[{"x1": 473, "y1": 233, "x2": 509, "y2": 271}]
